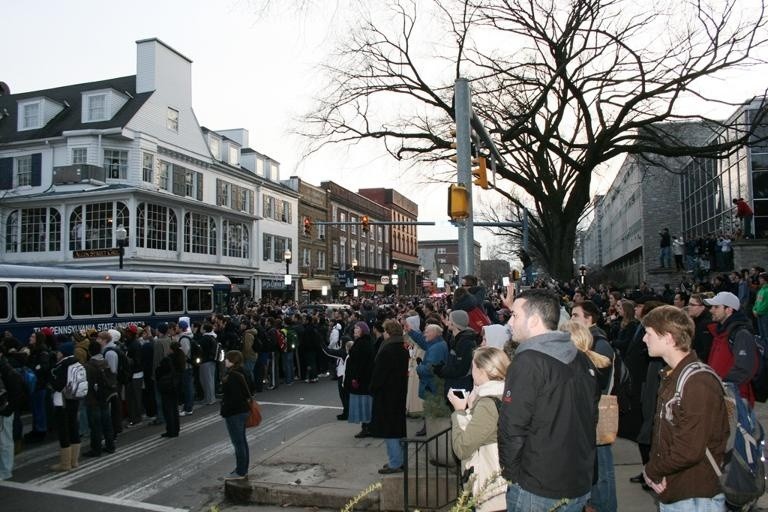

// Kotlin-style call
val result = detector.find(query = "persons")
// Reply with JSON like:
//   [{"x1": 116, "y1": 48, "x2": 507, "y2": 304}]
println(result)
[{"x1": 733, "y1": 198, "x2": 753, "y2": 239}]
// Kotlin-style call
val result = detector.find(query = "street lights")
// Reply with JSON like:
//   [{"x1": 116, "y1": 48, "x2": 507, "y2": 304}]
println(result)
[
  {"x1": 391, "y1": 263, "x2": 398, "y2": 295},
  {"x1": 350, "y1": 258, "x2": 360, "y2": 299},
  {"x1": 578, "y1": 261, "x2": 586, "y2": 286},
  {"x1": 419, "y1": 267, "x2": 424, "y2": 296},
  {"x1": 282, "y1": 249, "x2": 293, "y2": 305},
  {"x1": 454, "y1": 269, "x2": 460, "y2": 289},
  {"x1": 439, "y1": 268, "x2": 444, "y2": 291},
  {"x1": 113, "y1": 223, "x2": 129, "y2": 272}
]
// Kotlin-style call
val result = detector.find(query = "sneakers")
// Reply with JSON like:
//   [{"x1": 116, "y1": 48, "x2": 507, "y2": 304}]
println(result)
[
  {"x1": 84, "y1": 446, "x2": 101, "y2": 457},
  {"x1": 199, "y1": 396, "x2": 218, "y2": 406},
  {"x1": 178, "y1": 409, "x2": 195, "y2": 417},
  {"x1": 225, "y1": 469, "x2": 249, "y2": 480},
  {"x1": 378, "y1": 463, "x2": 406, "y2": 474},
  {"x1": 101, "y1": 440, "x2": 116, "y2": 453},
  {"x1": 336, "y1": 413, "x2": 349, "y2": 422},
  {"x1": 353, "y1": 426, "x2": 374, "y2": 439},
  {"x1": 161, "y1": 431, "x2": 179, "y2": 437},
  {"x1": 267, "y1": 375, "x2": 320, "y2": 390}
]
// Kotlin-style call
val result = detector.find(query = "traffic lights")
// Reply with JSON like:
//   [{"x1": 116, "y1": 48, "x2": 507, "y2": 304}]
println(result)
[
  {"x1": 471, "y1": 155, "x2": 487, "y2": 190},
  {"x1": 361, "y1": 215, "x2": 369, "y2": 232},
  {"x1": 303, "y1": 216, "x2": 311, "y2": 235},
  {"x1": 446, "y1": 124, "x2": 457, "y2": 162},
  {"x1": 445, "y1": 183, "x2": 469, "y2": 219},
  {"x1": 511, "y1": 269, "x2": 519, "y2": 281}
]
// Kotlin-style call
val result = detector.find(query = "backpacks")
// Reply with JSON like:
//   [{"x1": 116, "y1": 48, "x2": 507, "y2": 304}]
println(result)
[
  {"x1": 727, "y1": 323, "x2": 768, "y2": 404},
  {"x1": 166, "y1": 372, "x2": 195, "y2": 402},
  {"x1": 274, "y1": 330, "x2": 287, "y2": 354},
  {"x1": 285, "y1": 329, "x2": 298, "y2": 352},
  {"x1": 2, "y1": 363, "x2": 31, "y2": 412},
  {"x1": 594, "y1": 334, "x2": 634, "y2": 416},
  {"x1": 248, "y1": 331, "x2": 272, "y2": 354},
  {"x1": 178, "y1": 335, "x2": 203, "y2": 369},
  {"x1": 209, "y1": 336, "x2": 226, "y2": 364},
  {"x1": 59, "y1": 362, "x2": 89, "y2": 401},
  {"x1": 103, "y1": 347, "x2": 134, "y2": 385},
  {"x1": 676, "y1": 363, "x2": 766, "y2": 512}
]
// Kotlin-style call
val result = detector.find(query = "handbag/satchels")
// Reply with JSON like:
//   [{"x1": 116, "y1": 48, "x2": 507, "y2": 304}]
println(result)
[
  {"x1": 595, "y1": 394, "x2": 620, "y2": 447},
  {"x1": 464, "y1": 441, "x2": 508, "y2": 512},
  {"x1": 86, "y1": 374, "x2": 104, "y2": 401},
  {"x1": 245, "y1": 397, "x2": 262, "y2": 428}
]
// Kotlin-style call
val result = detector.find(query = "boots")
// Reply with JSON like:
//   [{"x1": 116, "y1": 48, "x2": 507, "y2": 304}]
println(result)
[{"x1": 48, "y1": 442, "x2": 81, "y2": 471}]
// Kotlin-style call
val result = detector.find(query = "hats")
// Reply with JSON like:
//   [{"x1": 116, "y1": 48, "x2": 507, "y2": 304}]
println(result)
[
  {"x1": 40, "y1": 327, "x2": 53, "y2": 336},
  {"x1": 178, "y1": 321, "x2": 188, "y2": 330},
  {"x1": 356, "y1": 321, "x2": 370, "y2": 336},
  {"x1": 702, "y1": 291, "x2": 741, "y2": 312},
  {"x1": 107, "y1": 329, "x2": 122, "y2": 343},
  {"x1": 122, "y1": 325, "x2": 139, "y2": 335},
  {"x1": 449, "y1": 309, "x2": 471, "y2": 332}
]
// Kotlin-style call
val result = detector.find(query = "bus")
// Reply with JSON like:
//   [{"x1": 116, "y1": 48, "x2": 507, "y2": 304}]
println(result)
[{"x1": 0, "y1": 263, "x2": 233, "y2": 355}]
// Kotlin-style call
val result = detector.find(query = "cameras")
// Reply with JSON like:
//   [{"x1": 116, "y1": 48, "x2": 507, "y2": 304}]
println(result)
[{"x1": 450, "y1": 389, "x2": 466, "y2": 400}]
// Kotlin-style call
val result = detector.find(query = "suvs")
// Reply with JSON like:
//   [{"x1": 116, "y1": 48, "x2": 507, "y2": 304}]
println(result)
[{"x1": 299, "y1": 304, "x2": 351, "y2": 319}]
